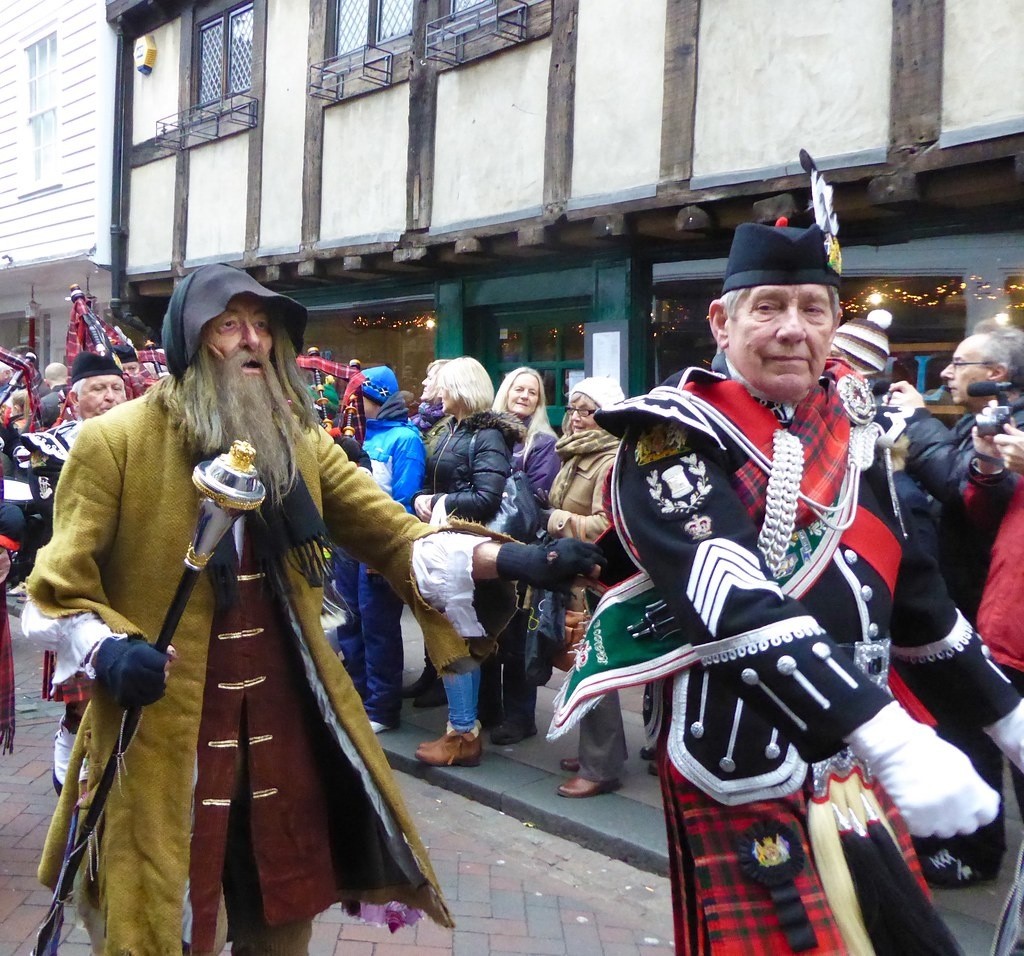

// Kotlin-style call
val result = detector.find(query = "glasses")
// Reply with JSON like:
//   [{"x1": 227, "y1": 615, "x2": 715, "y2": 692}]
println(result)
[
  {"x1": 951, "y1": 360, "x2": 993, "y2": 366},
  {"x1": 565, "y1": 406, "x2": 596, "y2": 417}
]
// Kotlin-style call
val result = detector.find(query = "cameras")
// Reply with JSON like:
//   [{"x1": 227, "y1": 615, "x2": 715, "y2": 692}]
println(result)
[{"x1": 975, "y1": 405, "x2": 1015, "y2": 437}]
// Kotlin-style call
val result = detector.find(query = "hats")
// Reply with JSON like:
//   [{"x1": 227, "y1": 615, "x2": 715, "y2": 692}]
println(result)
[
  {"x1": 72, "y1": 352, "x2": 124, "y2": 383},
  {"x1": 161, "y1": 264, "x2": 308, "y2": 377},
  {"x1": 720, "y1": 149, "x2": 844, "y2": 295},
  {"x1": 360, "y1": 366, "x2": 398, "y2": 406},
  {"x1": 828, "y1": 308, "x2": 892, "y2": 375},
  {"x1": 112, "y1": 345, "x2": 139, "y2": 363}
]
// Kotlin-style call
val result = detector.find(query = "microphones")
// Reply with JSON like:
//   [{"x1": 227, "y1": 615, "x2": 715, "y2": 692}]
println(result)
[{"x1": 965, "y1": 376, "x2": 1018, "y2": 399}]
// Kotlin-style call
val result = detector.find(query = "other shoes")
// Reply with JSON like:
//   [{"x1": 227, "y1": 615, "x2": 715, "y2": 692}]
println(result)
[{"x1": 491, "y1": 717, "x2": 538, "y2": 744}]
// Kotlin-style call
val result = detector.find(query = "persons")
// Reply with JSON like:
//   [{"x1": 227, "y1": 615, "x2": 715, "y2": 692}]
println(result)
[
  {"x1": 331, "y1": 357, "x2": 660, "y2": 798},
  {"x1": 21, "y1": 263, "x2": 608, "y2": 954},
  {"x1": 0, "y1": 343, "x2": 171, "y2": 796},
  {"x1": 547, "y1": 223, "x2": 1024, "y2": 956}
]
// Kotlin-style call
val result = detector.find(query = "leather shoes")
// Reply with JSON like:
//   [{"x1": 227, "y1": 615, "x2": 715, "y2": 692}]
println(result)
[
  {"x1": 558, "y1": 776, "x2": 621, "y2": 798},
  {"x1": 560, "y1": 758, "x2": 580, "y2": 771},
  {"x1": 402, "y1": 679, "x2": 448, "y2": 708}
]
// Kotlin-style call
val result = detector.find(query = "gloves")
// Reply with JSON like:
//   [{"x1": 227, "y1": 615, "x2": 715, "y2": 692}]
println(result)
[
  {"x1": 848, "y1": 699, "x2": 1001, "y2": 837},
  {"x1": 984, "y1": 696, "x2": 1024, "y2": 774},
  {"x1": 496, "y1": 537, "x2": 608, "y2": 590}
]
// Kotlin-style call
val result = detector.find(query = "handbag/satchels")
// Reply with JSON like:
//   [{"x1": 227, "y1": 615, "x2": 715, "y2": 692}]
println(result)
[{"x1": 483, "y1": 471, "x2": 542, "y2": 542}]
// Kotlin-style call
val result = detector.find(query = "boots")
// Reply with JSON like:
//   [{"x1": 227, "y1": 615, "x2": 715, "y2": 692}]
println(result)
[
  {"x1": 415, "y1": 726, "x2": 483, "y2": 766},
  {"x1": 419, "y1": 720, "x2": 482, "y2": 750}
]
